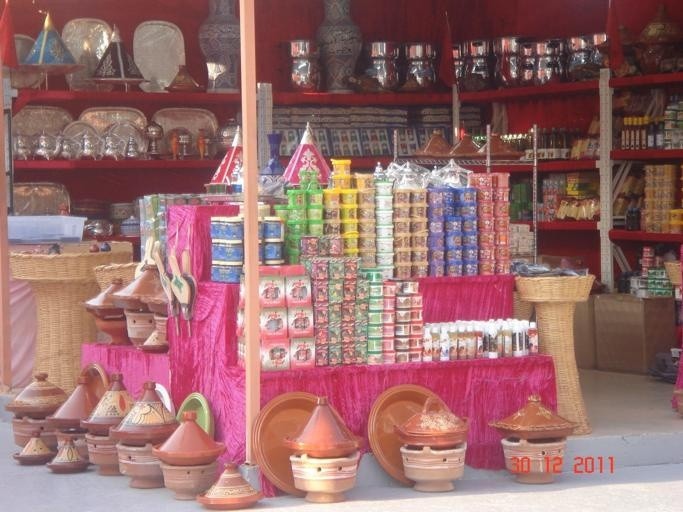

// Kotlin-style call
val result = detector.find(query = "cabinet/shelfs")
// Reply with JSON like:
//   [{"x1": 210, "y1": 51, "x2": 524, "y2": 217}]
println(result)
[{"x1": 1, "y1": 66, "x2": 683, "y2": 494}]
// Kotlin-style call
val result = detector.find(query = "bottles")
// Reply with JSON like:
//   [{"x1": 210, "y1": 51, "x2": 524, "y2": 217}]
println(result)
[
  {"x1": 504, "y1": 127, "x2": 572, "y2": 160},
  {"x1": 622, "y1": 114, "x2": 663, "y2": 150},
  {"x1": 424, "y1": 318, "x2": 538, "y2": 361},
  {"x1": 626, "y1": 207, "x2": 640, "y2": 230}
]
